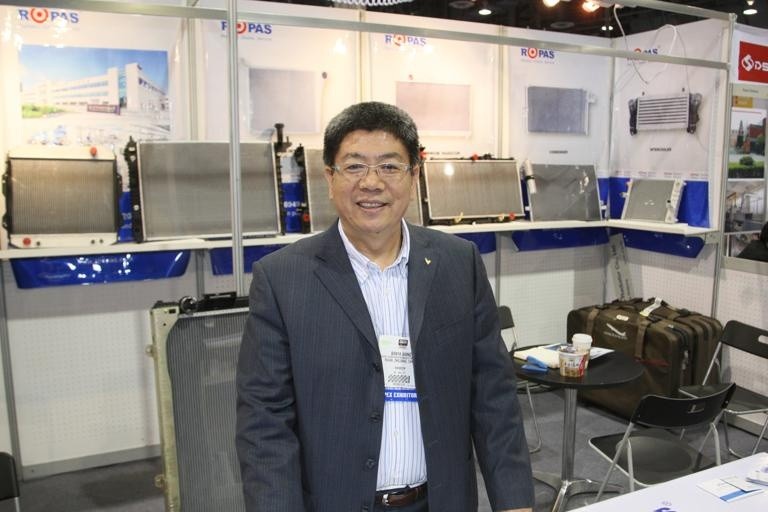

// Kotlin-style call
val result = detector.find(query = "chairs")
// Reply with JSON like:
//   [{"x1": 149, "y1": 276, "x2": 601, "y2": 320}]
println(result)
[
  {"x1": 492, "y1": 307, "x2": 560, "y2": 455},
  {"x1": 587, "y1": 320, "x2": 768, "y2": 500}
]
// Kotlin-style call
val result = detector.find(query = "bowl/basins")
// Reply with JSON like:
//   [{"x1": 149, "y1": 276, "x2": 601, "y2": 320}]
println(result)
[{"x1": 559, "y1": 349, "x2": 589, "y2": 377}]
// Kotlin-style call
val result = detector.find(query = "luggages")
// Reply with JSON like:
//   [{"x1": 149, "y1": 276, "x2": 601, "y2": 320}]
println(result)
[{"x1": 558, "y1": 295, "x2": 726, "y2": 420}]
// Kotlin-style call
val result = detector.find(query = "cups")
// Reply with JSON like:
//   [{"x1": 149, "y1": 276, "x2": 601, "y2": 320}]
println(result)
[{"x1": 571, "y1": 333, "x2": 592, "y2": 369}]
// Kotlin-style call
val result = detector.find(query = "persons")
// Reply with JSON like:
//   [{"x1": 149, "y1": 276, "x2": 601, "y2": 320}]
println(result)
[{"x1": 233, "y1": 100, "x2": 537, "y2": 512}]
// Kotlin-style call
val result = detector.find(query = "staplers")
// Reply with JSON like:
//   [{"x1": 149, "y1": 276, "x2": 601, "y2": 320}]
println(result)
[{"x1": 521, "y1": 356, "x2": 548, "y2": 372}]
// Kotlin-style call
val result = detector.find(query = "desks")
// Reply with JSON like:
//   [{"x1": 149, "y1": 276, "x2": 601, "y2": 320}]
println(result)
[
  {"x1": 506, "y1": 342, "x2": 647, "y2": 512},
  {"x1": 558, "y1": 450, "x2": 767, "y2": 511}
]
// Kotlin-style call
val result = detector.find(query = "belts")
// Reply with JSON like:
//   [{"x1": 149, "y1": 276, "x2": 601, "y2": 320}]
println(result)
[{"x1": 374, "y1": 482, "x2": 426, "y2": 507}]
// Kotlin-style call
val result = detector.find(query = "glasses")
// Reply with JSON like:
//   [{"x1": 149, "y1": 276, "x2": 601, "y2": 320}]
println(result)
[{"x1": 330, "y1": 162, "x2": 413, "y2": 179}]
